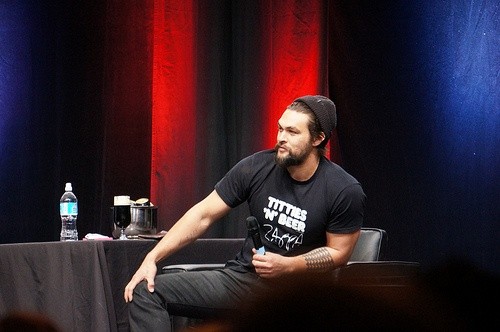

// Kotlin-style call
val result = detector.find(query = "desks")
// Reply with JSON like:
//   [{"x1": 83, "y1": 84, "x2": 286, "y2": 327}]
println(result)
[{"x1": 0, "y1": 238, "x2": 245, "y2": 332}]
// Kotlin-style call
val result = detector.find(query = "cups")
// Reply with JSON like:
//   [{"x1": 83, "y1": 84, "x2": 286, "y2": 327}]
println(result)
[{"x1": 131, "y1": 197, "x2": 151, "y2": 206}]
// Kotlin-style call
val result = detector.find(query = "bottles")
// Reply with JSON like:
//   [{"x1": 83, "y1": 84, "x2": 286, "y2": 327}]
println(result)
[{"x1": 59, "y1": 182, "x2": 78, "y2": 242}]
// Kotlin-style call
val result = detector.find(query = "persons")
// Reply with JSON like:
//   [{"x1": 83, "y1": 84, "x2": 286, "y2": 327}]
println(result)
[{"x1": 124, "y1": 94, "x2": 367, "y2": 332}]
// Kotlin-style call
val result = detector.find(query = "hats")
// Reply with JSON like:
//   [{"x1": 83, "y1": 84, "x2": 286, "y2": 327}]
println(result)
[{"x1": 294, "y1": 95, "x2": 336, "y2": 143}]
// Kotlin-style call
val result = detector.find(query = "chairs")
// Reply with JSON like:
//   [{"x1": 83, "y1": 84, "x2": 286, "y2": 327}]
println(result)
[{"x1": 163, "y1": 228, "x2": 418, "y2": 332}]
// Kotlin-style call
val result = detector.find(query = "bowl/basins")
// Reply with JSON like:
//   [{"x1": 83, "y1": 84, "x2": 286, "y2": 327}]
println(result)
[{"x1": 113, "y1": 206, "x2": 158, "y2": 235}]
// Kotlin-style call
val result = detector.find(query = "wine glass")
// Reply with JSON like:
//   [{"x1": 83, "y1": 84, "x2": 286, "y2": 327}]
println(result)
[{"x1": 114, "y1": 196, "x2": 131, "y2": 241}]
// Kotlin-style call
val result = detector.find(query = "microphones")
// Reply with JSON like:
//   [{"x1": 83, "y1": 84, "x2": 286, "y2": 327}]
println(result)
[{"x1": 245, "y1": 215, "x2": 264, "y2": 256}]
